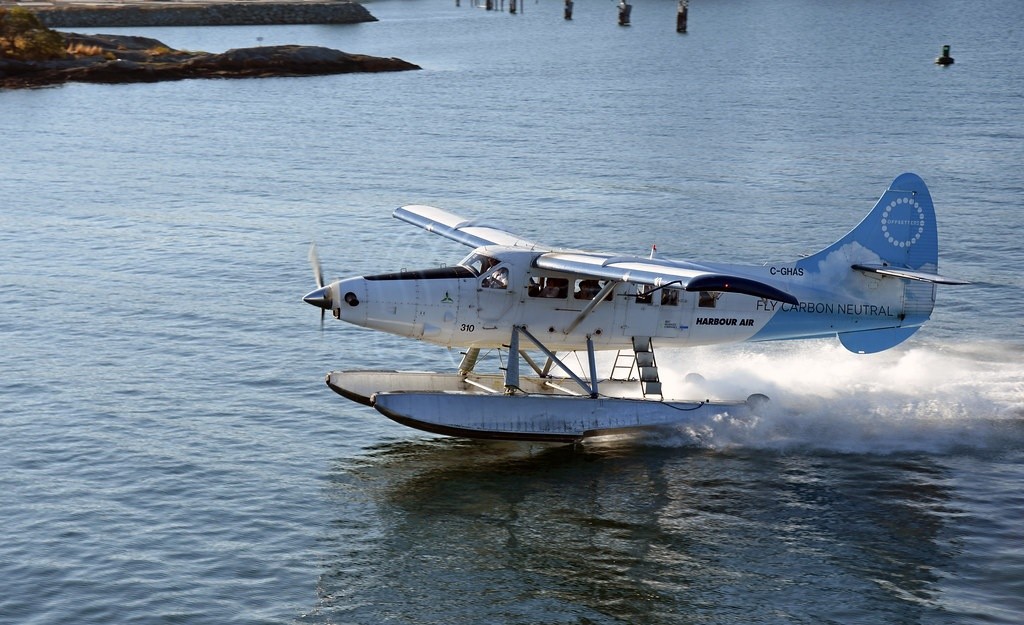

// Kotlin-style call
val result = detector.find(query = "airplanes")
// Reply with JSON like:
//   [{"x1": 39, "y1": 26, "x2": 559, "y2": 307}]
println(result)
[{"x1": 300, "y1": 166, "x2": 974, "y2": 456}]
